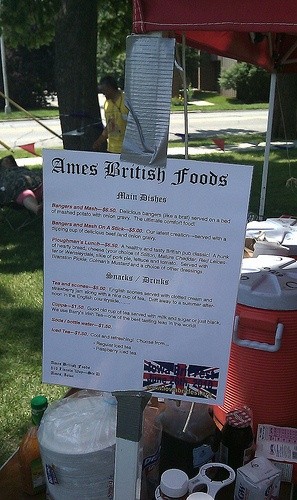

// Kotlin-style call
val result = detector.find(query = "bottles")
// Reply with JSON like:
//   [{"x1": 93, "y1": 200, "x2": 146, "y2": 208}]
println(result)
[
  {"x1": 214, "y1": 409, "x2": 255, "y2": 480},
  {"x1": 154, "y1": 468, "x2": 215, "y2": 500},
  {"x1": 30, "y1": 395, "x2": 49, "y2": 427},
  {"x1": 159, "y1": 397, "x2": 220, "y2": 492}
]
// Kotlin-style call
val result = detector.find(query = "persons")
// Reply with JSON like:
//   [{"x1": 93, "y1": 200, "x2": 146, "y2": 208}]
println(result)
[
  {"x1": 0, "y1": 157, "x2": 43, "y2": 215},
  {"x1": 92, "y1": 75, "x2": 129, "y2": 153}
]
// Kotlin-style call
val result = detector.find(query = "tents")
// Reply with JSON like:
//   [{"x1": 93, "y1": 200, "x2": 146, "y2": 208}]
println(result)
[{"x1": 131, "y1": 0, "x2": 297, "y2": 220}]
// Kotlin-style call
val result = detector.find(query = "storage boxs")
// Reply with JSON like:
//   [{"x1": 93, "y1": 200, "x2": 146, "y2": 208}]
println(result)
[
  {"x1": 234, "y1": 456, "x2": 282, "y2": 500},
  {"x1": 254, "y1": 424, "x2": 297, "y2": 482}
]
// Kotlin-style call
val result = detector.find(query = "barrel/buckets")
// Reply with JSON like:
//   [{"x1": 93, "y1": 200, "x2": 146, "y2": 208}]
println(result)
[
  {"x1": 211, "y1": 254, "x2": 297, "y2": 437},
  {"x1": 243, "y1": 216, "x2": 297, "y2": 258},
  {"x1": 38, "y1": 392, "x2": 116, "y2": 500}
]
